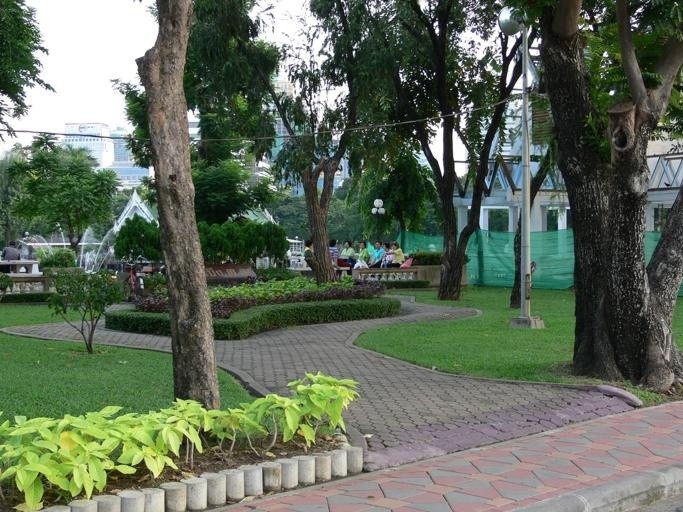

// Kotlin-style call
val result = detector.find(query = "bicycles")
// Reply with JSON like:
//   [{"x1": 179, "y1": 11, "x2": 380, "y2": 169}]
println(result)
[{"x1": 120, "y1": 255, "x2": 146, "y2": 301}]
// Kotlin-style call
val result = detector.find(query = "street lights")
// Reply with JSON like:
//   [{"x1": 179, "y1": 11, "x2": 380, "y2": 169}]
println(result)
[
  {"x1": 371, "y1": 199, "x2": 385, "y2": 240},
  {"x1": 497, "y1": 7, "x2": 545, "y2": 330}
]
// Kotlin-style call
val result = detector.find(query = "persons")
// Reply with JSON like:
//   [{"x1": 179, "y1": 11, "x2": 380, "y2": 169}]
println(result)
[
  {"x1": 328, "y1": 239, "x2": 406, "y2": 268},
  {"x1": 304, "y1": 239, "x2": 313, "y2": 253},
  {"x1": 0, "y1": 241, "x2": 21, "y2": 273}
]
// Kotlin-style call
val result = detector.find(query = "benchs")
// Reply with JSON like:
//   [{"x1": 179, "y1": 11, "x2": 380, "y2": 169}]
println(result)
[
  {"x1": 0, "y1": 259, "x2": 45, "y2": 291},
  {"x1": 287, "y1": 264, "x2": 350, "y2": 281}
]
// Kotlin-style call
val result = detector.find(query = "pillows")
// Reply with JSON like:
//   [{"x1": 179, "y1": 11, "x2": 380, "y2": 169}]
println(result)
[{"x1": 401, "y1": 256, "x2": 413, "y2": 269}]
